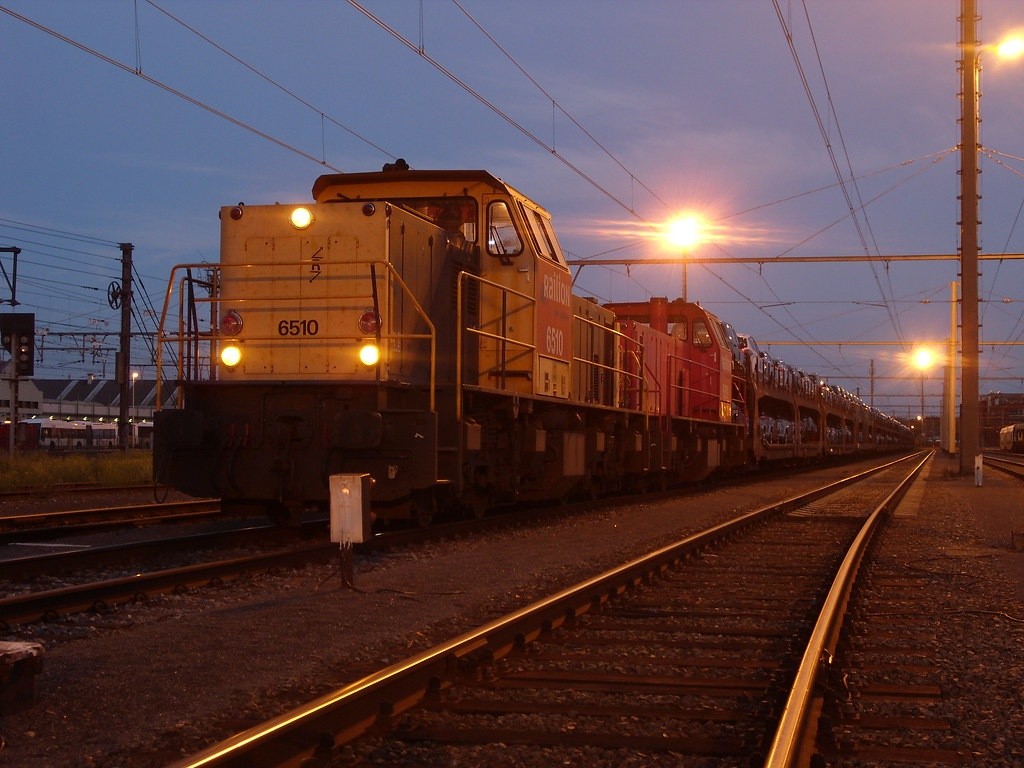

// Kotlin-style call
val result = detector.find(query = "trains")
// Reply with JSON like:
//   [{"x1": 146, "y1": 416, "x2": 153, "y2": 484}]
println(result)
[
  {"x1": 146, "y1": 161, "x2": 929, "y2": 538},
  {"x1": 1000, "y1": 423, "x2": 1024, "y2": 452}
]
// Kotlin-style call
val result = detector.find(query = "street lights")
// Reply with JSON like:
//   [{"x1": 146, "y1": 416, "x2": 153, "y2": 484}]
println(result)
[
  {"x1": 912, "y1": 347, "x2": 931, "y2": 433},
  {"x1": 665, "y1": 208, "x2": 712, "y2": 302},
  {"x1": 133, "y1": 372, "x2": 138, "y2": 421}
]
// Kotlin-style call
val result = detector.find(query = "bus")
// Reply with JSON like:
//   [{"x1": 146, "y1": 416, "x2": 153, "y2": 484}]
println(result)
[
  {"x1": 20, "y1": 420, "x2": 139, "y2": 449},
  {"x1": 138, "y1": 421, "x2": 154, "y2": 449}
]
